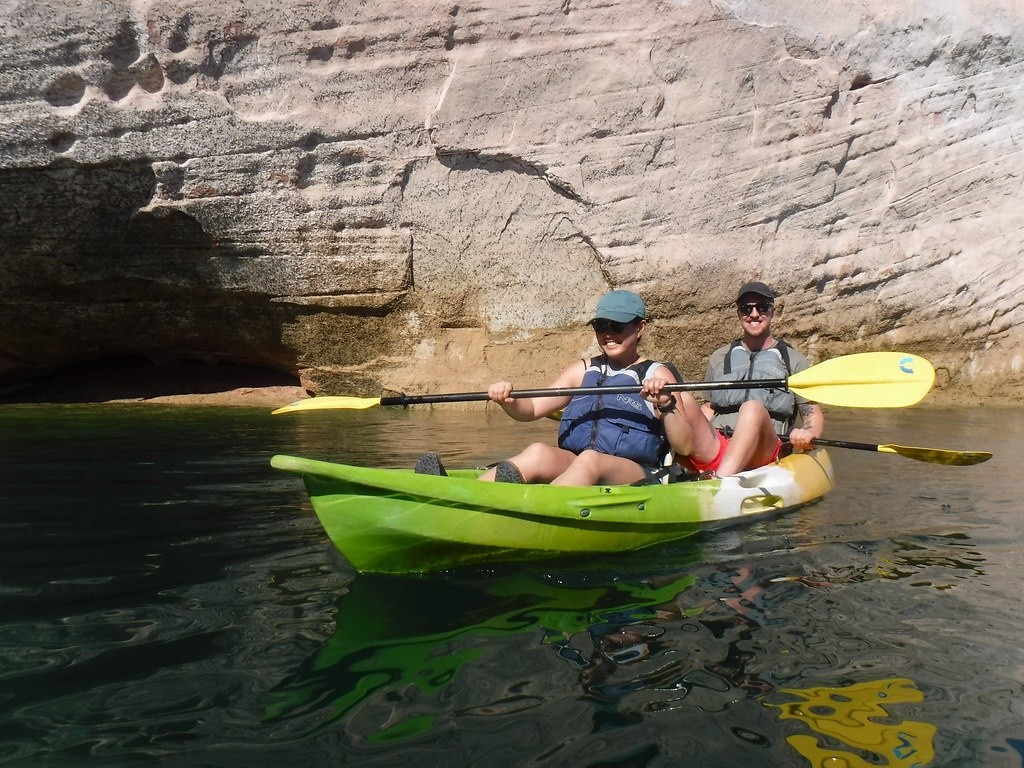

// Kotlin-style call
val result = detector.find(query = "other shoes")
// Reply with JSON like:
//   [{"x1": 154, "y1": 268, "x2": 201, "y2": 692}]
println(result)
[
  {"x1": 495, "y1": 459, "x2": 525, "y2": 483},
  {"x1": 415, "y1": 454, "x2": 447, "y2": 476}
]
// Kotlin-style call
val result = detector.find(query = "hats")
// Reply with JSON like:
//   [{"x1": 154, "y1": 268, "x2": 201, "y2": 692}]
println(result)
[
  {"x1": 585, "y1": 291, "x2": 645, "y2": 327},
  {"x1": 735, "y1": 282, "x2": 774, "y2": 307}
]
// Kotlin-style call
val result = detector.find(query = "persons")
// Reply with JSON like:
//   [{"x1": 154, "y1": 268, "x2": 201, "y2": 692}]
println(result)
[
  {"x1": 414, "y1": 289, "x2": 696, "y2": 487},
  {"x1": 673, "y1": 280, "x2": 824, "y2": 480}
]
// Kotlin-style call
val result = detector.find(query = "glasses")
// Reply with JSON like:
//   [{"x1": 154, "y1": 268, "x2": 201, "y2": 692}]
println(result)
[
  {"x1": 738, "y1": 302, "x2": 772, "y2": 317},
  {"x1": 592, "y1": 321, "x2": 627, "y2": 334}
]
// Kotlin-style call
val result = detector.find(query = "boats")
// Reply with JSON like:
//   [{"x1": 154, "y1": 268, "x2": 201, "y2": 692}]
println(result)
[{"x1": 270, "y1": 447, "x2": 840, "y2": 588}]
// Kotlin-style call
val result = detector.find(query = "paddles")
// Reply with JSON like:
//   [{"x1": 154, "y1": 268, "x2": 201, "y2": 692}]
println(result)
[
  {"x1": 268, "y1": 352, "x2": 937, "y2": 410},
  {"x1": 708, "y1": 426, "x2": 994, "y2": 467}
]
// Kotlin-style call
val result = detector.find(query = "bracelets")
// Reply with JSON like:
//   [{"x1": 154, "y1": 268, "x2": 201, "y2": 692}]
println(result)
[{"x1": 657, "y1": 395, "x2": 677, "y2": 412}]
